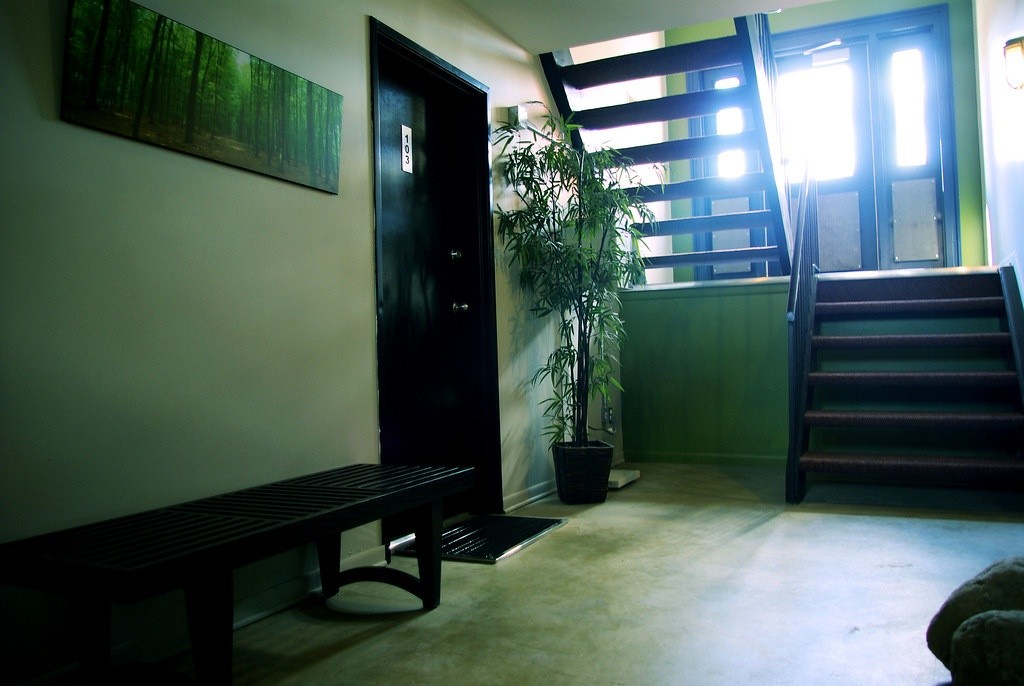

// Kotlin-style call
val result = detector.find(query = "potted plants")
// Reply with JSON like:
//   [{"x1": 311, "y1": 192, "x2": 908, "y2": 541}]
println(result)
[{"x1": 489, "y1": 98, "x2": 661, "y2": 506}]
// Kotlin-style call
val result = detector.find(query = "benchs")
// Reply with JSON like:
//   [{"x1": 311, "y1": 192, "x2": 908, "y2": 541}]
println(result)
[{"x1": 0, "y1": 462, "x2": 475, "y2": 686}]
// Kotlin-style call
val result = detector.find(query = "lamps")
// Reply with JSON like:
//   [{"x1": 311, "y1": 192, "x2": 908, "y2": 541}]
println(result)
[{"x1": 1003, "y1": 34, "x2": 1024, "y2": 89}]
